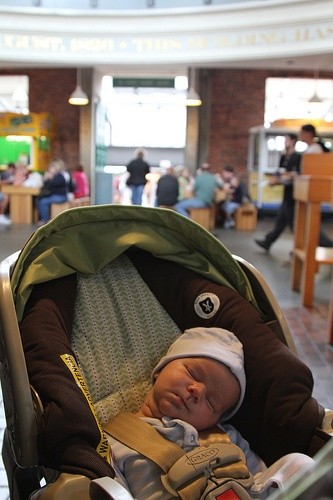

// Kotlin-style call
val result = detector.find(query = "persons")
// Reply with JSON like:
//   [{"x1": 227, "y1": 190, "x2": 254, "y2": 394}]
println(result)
[
  {"x1": 103, "y1": 326, "x2": 277, "y2": 500},
  {"x1": 112, "y1": 151, "x2": 243, "y2": 230},
  {"x1": 0, "y1": 154, "x2": 90, "y2": 227},
  {"x1": 253, "y1": 124, "x2": 333, "y2": 251}
]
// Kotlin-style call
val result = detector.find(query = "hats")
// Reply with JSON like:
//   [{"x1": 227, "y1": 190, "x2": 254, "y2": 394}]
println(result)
[{"x1": 151, "y1": 327, "x2": 246, "y2": 421}]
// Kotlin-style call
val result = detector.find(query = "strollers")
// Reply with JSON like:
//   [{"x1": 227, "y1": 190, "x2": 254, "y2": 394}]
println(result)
[{"x1": 0, "y1": 203, "x2": 331, "y2": 495}]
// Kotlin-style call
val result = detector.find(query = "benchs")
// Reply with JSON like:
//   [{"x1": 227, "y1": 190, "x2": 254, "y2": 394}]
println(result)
[
  {"x1": 233, "y1": 203, "x2": 258, "y2": 231},
  {"x1": 50, "y1": 196, "x2": 92, "y2": 220},
  {"x1": 186, "y1": 206, "x2": 215, "y2": 231}
]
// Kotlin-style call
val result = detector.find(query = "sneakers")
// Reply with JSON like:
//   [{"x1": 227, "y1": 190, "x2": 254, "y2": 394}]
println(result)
[{"x1": 0, "y1": 215, "x2": 11, "y2": 224}]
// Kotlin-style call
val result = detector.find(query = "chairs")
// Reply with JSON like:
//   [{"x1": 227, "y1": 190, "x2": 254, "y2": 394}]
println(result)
[{"x1": 301, "y1": 153, "x2": 333, "y2": 176}]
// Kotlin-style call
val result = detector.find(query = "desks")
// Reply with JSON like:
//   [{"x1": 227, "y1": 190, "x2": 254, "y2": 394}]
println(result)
[
  {"x1": 291, "y1": 176, "x2": 333, "y2": 308},
  {"x1": 1, "y1": 186, "x2": 41, "y2": 225},
  {"x1": 215, "y1": 189, "x2": 235, "y2": 203}
]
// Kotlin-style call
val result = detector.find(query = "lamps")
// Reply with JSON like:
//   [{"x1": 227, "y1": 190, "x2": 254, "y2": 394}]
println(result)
[
  {"x1": 68, "y1": 67, "x2": 89, "y2": 105},
  {"x1": 184, "y1": 67, "x2": 202, "y2": 107},
  {"x1": 307, "y1": 69, "x2": 324, "y2": 103}
]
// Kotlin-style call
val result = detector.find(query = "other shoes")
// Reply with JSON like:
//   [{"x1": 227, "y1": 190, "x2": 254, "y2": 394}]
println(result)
[
  {"x1": 255, "y1": 238, "x2": 270, "y2": 250},
  {"x1": 225, "y1": 219, "x2": 235, "y2": 229}
]
